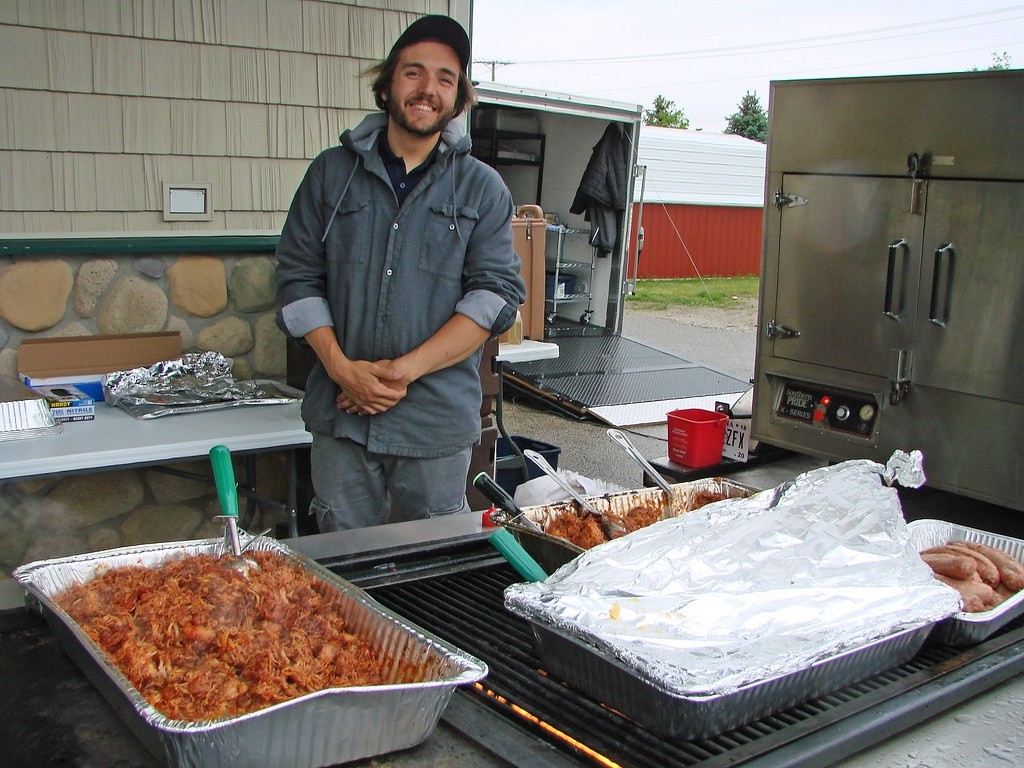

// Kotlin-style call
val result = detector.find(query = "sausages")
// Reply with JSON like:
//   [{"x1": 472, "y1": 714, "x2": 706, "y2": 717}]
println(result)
[{"x1": 917, "y1": 538, "x2": 1024, "y2": 613}]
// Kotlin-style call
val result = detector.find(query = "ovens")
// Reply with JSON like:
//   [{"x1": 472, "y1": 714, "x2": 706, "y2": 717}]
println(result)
[{"x1": 749, "y1": 70, "x2": 1024, "y2": 513}]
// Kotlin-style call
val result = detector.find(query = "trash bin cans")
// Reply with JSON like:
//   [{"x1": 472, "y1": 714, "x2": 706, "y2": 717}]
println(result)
[{"x1": 495, "y1": 435, "x2": 561, "y2": 499}]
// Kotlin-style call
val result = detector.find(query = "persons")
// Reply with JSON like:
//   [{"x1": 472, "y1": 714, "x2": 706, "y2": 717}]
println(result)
[{"x1": 275, "y1": 15, "x2": 527, "y2": 534}]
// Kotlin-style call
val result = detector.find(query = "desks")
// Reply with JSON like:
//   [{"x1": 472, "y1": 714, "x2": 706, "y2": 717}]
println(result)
[
  {"x1": 0, "y1": 379, "x2": 313, "y2": 539},
  {"x1": 494, "y1": 339, "x2": 559, "y2": 486}
]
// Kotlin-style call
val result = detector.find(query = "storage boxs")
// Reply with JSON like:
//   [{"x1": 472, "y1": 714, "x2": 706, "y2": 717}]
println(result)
[
  {"x1": 274, "y1": 316, "x2": 562, "y2": 535},
  {"x1": 18, "y1": 330, "x2": 181, "y2": 399},
  {"x1": 906, "y1": 518, "x2": 1024, "y2": 642},
  {"x1": 470, "y1": 106, "x2": 541, "y2": 133},
  {"x1": 12, "y1": 532, "x2": 489, "y2": 768},
  {"x1": 31, "y1": 385, "x2": 95, "y2": 423},
  {"x1": 502, "y1": 578, "x2": 962, "y2": 743},
  {"x1": 486, "y1": 473, "x2": 768, "y2": 563}
]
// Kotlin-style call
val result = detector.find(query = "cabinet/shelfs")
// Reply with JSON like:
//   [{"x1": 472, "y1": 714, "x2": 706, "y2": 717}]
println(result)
[
  {"x1": 471, "y1": 129, "x2": 546, "y2": 207},
  {"x1": 546, "y1": 225, "x2": 598, "y2": 325}
]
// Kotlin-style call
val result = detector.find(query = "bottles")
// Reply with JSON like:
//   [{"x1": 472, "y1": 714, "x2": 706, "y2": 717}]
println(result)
[{"x1": 508, "y1": 312, "x2": 523, "y2": 345}]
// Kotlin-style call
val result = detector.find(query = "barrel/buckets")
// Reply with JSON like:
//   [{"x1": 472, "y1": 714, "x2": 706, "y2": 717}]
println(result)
[{"x1": 666, "y1": 408, "x2": 729, "y2": 468}]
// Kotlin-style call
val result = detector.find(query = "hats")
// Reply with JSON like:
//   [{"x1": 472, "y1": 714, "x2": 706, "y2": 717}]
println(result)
[{"x1": 388, "y1": 14, "x2": 470, "y2": 76}]
